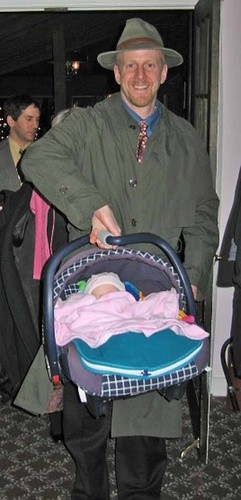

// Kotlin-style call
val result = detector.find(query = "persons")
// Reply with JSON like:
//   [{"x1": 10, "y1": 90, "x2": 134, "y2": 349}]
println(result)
[
  {"x1": 20, "y1": 19, "x2": 220, "y2": 500},
  {"x1": 83, "y1": 270, "x2": 126, "y2": 301},
  {"x1": 0, "y1": 92, "x2": 40, "y2": 192},
  {"x1": 51, "y1": 103, "x2": 89, "y2": 128}
]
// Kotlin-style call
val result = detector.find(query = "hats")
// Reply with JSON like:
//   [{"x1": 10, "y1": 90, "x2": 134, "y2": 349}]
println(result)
[
  {"x1": 83, "y1": 272, "x2": 125, "y2": 294},
  {"x1": 97, "y1": 19, "x2": 183, "y2": 69}
]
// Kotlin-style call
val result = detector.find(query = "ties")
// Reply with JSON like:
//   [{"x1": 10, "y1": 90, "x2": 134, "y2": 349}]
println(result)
[{"x1": 137, "y1": 120, "x2": 149, "y2": 165}]
